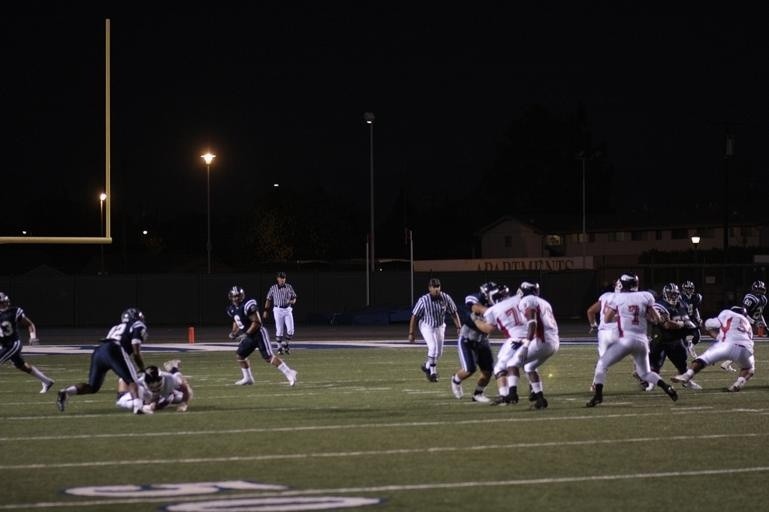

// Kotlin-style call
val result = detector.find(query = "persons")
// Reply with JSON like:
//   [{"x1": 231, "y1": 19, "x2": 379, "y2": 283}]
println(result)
[
  {"x1": 227, "y1": 286, "x2": 298, "y2": 387},
  {"x1": 0, "y1": 292, "x2": 55, "y2": 395},
  {"x1": 632, "y1": 281, "x2": 704, "y2": 390},
  {"x1": 262, "y1": 272, "x2": 297, "y2": 355},
  {"x1": 670, "y1": 306, "x2": 757, "y2": 393},
  {"x1": 720, "y1": 281, "x2": 767, "y2": 374},
  {"x1": 586, "y1": 278, "x2": 657, "y2": 392},
  {"x1": 408, "y1": 279, "x2": 462, "y2": 382},
  {"x1": 449, "y1": 279, "x2": 560, "y2": 410},
  {"x1": 117, "y1": 359, "x2": 193, "y2": 413},
  {"x1": 585, "y1": 274, "x2": 678, "y2": 408},
  {"x1": 56, "y1": 308, "x2": 148, "y2": 413}
]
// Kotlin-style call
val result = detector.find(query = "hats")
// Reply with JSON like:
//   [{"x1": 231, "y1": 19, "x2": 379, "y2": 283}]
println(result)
[
  {"x1": 427, "y1": 277, "x2": 441, "y2": 287},
  {"x1": 277, "y1": 272, "x2": 287, "y2": 278}
]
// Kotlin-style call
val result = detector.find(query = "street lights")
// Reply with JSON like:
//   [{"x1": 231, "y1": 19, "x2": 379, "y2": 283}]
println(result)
[
  {"x1": 689, "y1": 234, "x2": 702, "y2": 252},
  {"x1": 200, "y1": 153, "x2": 216, "y2": 274},
  {"x1": 574, "y1": 151, "x2": 590, "y2": 232},
  {"x1": 362, "y1": 112, "x2": 376, "y2": 273},
  {"x1": 98, "y1": 192, "x2": 107, "y2": 274},
  {"x1": 714, "y1": 119, "x2": 744, "y2": 263}
]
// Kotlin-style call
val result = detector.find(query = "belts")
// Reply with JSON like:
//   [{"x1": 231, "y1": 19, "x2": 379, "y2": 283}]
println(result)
[{"x1": 274, "y1": 304, "x2": 291, "y2": 308}]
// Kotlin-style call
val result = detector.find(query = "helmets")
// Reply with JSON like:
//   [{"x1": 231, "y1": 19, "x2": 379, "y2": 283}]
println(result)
[
  {"x1": 487, "y1": 282, "x2": 509, "y2": 306},
  {"x1": 520, "y1": 281, "x2": 540, "y2": 295},
  {"x1": 144, "y1": 366, "x2": 161, "y2": 392},
  {"x1": 619, "y1": 273, "x2": 638, "y2": 291},
  {"x1": 663, "y1": 283, "x2": 679, "y2": 306},
  {"x1": 743, "y1": 294, "x2": 760, "y2": 312},
  {"x1": 0, "y1": 291, "x2": 11, "y2": 313},
  {"x1": 119, "y1": 307, "x2": 145, "y2": 324},
  {"x1": 752, "y1": 279, "x2": 766, "y2": 297},
  {"x1": 682, "y1": 280, "x2": 695, "y2": 300},
  {"x1": 227, "y1": 285, "x2": 245, "y2": 300},
  {"x1": 614, "y1": 275, "x2": 624, "y2": 294},
  {"x1": 479, "y1": 281, "x2": 499, "y2": 300},
  {"x1": 731, "y1": 305, "x2": 748, "y2": 316}
]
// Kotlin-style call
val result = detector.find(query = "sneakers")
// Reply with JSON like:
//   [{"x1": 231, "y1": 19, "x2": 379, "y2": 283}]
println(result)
[
  {"x1": 289, "y1": 369, "x2": 299, "y2": 387},
  {"x1": 586, "y1": 397, "x2": 603, "y2": 408},
  {"x1": 685, "y1": 380, "x2": 702, "y2": 390},
  {"x1": 450, "y1": 373, "x2": 464, "y2": 401},
  {"x1": 426, "y1": 370, "x2": 439, "y2": 382},
  {"x1": 234, "y1": 377, "x2": 255, "y2": 386},
  {"x1": 39, "y1": 378, "x2": 56, "y2": 395},
  {"x1": 665, "y1": 387, "x2": 677, "y2": 400},
  {"x1": 671, "y1": 376, "x2": 688, "y2": 384},
  {"x1": 133, "y1": 398, "x2": 144, "y2": 415},
  {"x1": 721, "y1": 361, "x2": 737, "y2": 373},
  {"x1": 504, "y1": 395, "x2": 519, "y2": 404},
  {"x1": 56, "y1": 390, "x2": 68, "y2": 413},
  {"x1": 276, "y1": 347, "x2": 283, "y2": 355},
  {"x1": 727, "y1": 385, "x2": 739, "y2": 392},
  {"x1": 286, "y1": 345, "x2": 290, "y2": 355},
  {"x1": 492, "y1": 398, "x2": 507, "y2": 407},
  {"x1": 471, "y1": 393, "x2": 492, "y2": 404},
  {"x1": 421, "y1": 362, "x2": 430, "y2": 373},
  {"x1": 529, "y1": 400, "x2": 548, "y2": 411}
]
